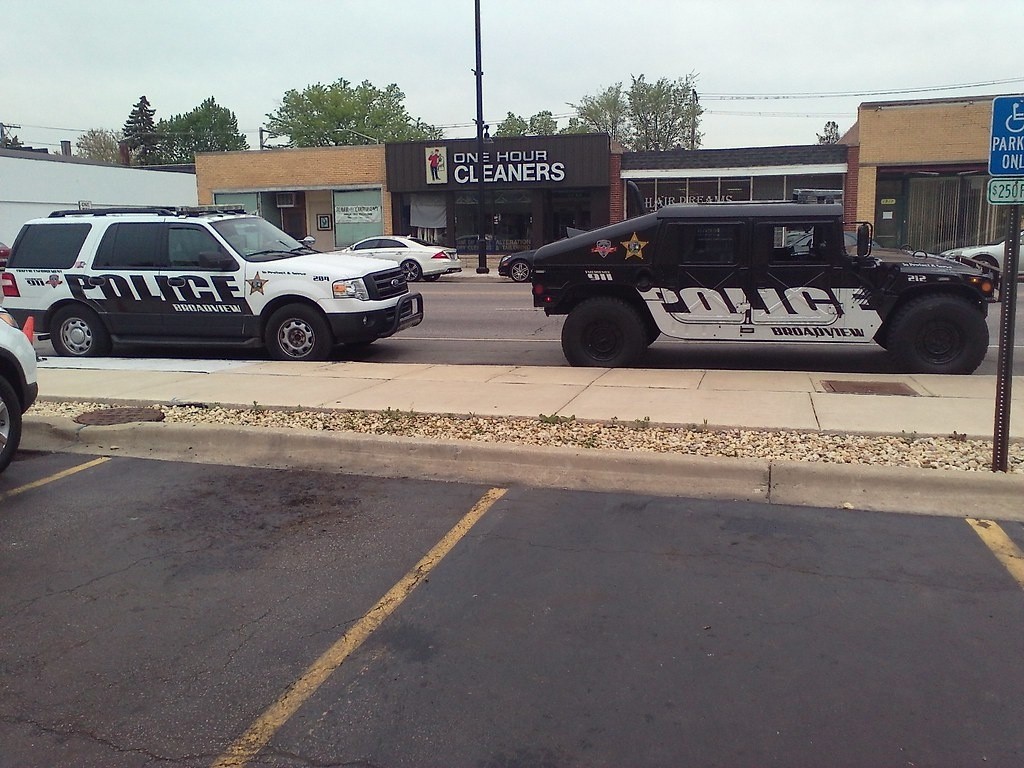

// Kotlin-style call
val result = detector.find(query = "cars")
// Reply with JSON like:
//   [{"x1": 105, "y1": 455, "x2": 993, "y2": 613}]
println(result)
[
  {"x1": 0, "y1": 319, "x2": 37, "y2": 472},
  {"x1": 456, "y1": 234, "x2": 502, "y2": 252},
  {"x1": 941, "y1": 230, "x2": 1024, "y2": 282},
  {"x1": 339, "y1": 236, "x2": 462, "y2": 282},
  {"x1": 497, "y1": 249, "x2": 538, "y2": 283}
]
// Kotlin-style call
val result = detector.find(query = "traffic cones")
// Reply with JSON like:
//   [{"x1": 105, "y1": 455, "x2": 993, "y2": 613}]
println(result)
[{"x1": 22, "y1": 315, "x2": 34, "y2": 345}]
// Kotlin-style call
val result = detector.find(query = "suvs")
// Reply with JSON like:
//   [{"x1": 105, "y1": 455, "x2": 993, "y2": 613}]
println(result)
[
  {"x1": 2, "y1": 206, "x2": 424, "y2": 361},
  {"x1": 531, "y1": 189, "x2": 1001, "y2": 375}
]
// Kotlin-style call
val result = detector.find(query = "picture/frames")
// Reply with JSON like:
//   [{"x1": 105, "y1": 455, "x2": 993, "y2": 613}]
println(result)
[{"x1": 316, "y1": 214, "x2": 333, "y2": 231}]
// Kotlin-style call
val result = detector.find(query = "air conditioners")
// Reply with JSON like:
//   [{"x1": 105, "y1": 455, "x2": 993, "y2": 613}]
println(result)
[{"x1": 276, "y1": 192, "x2": 302, "y2": 209}]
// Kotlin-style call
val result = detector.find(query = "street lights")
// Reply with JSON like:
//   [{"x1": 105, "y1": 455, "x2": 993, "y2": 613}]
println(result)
[
  {"x1": 333, "y1": 129, "x2": 378, "y2": 144},
  {"x1": 474, "y1": 124, "x2": 494, "y2": 273}
]
